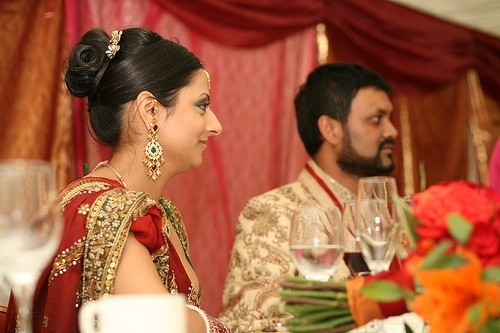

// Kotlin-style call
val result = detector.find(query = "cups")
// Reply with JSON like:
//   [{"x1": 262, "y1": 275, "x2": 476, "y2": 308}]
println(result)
[
  {"x1": 77, "y1": 294, "x2": 188, "y2": 333},
  {"x1": 340, "y1": 199, "x2": 389, "y2": 278},
  {"x1": 288, "y1": 204, "x2": 343, "y2": 282},
  {"x1": 356, "y1": 177, "x2": 402, "y2": 276}
]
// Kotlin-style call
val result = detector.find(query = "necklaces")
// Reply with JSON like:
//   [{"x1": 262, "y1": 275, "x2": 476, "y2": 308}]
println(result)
[{"x1": 90, "y1": 159, "x2": 170, "y2": 237}]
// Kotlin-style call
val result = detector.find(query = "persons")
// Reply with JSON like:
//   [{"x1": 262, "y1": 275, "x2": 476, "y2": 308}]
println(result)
[
  {"x1": 210, "y1": 62, "x2": 414, "y2": 332},
  {"x1": 3, "y1": 27, "x2": 222, "y2": 332}
]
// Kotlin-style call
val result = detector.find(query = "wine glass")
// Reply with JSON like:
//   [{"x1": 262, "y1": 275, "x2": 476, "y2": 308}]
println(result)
[{"x1": 0, "y1": 160, "x2": 63, "y2": 333}]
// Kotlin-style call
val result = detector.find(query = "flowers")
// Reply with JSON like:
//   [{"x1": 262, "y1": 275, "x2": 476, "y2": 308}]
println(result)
[{"x1": 279, "y1": 135, "x2": 500, "y2": 331}]
